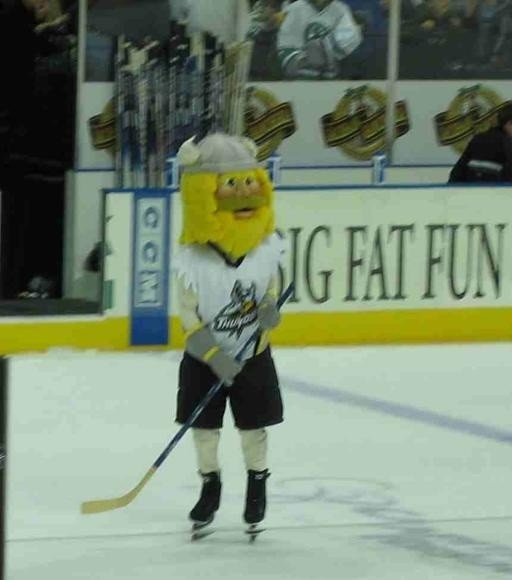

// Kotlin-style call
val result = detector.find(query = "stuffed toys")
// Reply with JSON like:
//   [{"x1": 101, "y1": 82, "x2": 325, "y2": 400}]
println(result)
[{"x1": 171, "y1": 134, "x2": 286, "y2": 525}]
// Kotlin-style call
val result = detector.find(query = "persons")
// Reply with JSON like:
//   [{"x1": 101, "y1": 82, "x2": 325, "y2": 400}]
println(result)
[
  {"x1": 5, "y1": 2, "x2": 79, "y2": 298},
  {"x1": 80, "y1": 1, "x2": 512, "y2": 82},
  {"x1": 445, "y1": 99, "x2": 511, "y2": 184}
]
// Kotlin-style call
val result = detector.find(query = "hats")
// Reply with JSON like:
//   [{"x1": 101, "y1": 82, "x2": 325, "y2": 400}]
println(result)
[{"x1": 498, "y1": 104, "x2": 512, "y2": 124}]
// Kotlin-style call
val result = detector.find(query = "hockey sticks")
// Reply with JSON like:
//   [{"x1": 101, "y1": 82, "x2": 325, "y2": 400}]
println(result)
[
  {"x1": 82, "y1": 281, "x2": 297, "y2": 514},
  {"x1": 113, "y1": 29, "x2": 250, "y2": 187}
]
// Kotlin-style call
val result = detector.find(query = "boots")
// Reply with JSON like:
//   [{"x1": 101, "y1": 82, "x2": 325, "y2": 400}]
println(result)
[
  {"x1": 189, "y1": 469, "x2": 222, "y2": 522},
  {"x1": 244, "y1": 469, "x2": 272, "y2": 523}
]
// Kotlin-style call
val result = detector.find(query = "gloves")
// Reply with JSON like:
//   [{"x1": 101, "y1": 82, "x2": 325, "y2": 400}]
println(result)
[
  {"x1": 258, "y1": 293, "x2": 281, "y2": 329},
  {"x1": 185, "y1": 323, "x2": 238, "y2": 380}
]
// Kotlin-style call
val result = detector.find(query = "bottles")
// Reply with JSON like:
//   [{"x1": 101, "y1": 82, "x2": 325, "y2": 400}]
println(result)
[
  {"x1": 372, "y1": 151, "x2": 386, "y2": 188},
  {"x1": 164, "y1": 153, "x2": 180, "y2": 191},
  {"x1": 266, "y1": 154, "x2": 281, "y2": 186}
]
